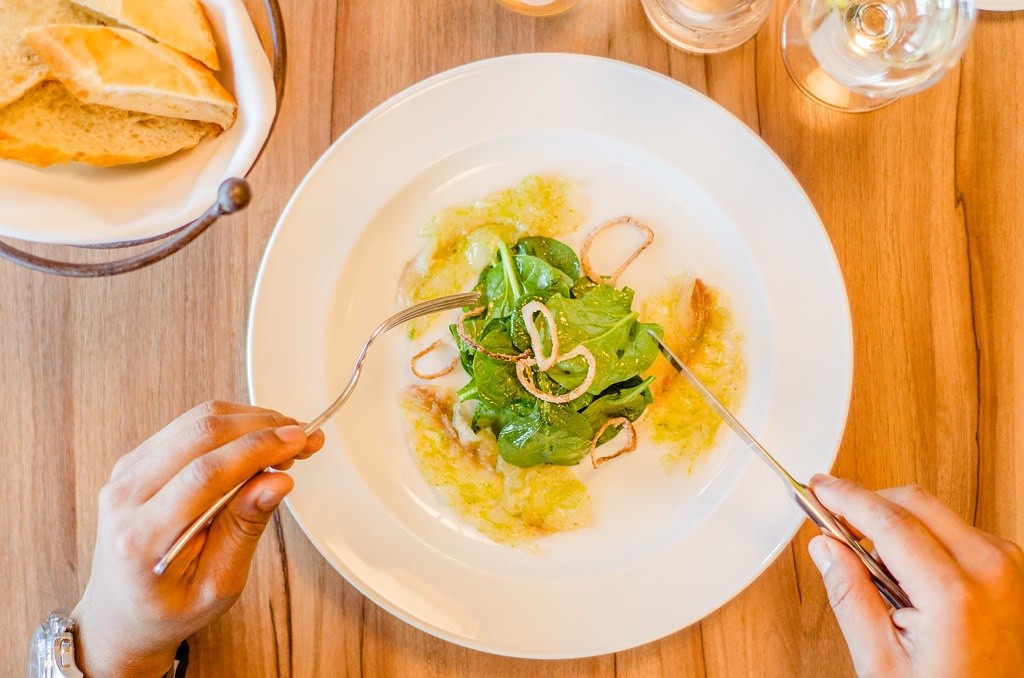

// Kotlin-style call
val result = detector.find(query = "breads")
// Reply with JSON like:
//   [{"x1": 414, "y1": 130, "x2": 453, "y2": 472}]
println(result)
[{"x1": 0, "y1": 0, "x2": 237, "y2": 168}]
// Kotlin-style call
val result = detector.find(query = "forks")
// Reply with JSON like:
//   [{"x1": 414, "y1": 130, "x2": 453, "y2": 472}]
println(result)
[{"x1": 153, "y1": 292, "x2": 482, "y2": 576}]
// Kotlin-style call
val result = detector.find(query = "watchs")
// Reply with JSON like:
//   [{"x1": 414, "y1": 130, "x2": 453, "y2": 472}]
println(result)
[{"x1": 28, "y1": 605, "x2": 191, "y2": 678}]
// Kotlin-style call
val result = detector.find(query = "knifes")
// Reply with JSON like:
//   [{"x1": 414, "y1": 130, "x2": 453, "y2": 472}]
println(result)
[{"x1": 647, "y1": 328, "x2": 914, "y2": 611}]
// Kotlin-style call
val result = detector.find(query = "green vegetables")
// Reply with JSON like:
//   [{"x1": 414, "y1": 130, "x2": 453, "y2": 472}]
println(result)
[{"x1": 449, "y1": 237, "x2": 665, "y2": 467}]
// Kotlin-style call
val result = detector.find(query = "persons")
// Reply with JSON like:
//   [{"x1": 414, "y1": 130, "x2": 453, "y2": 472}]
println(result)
[{"x1": 27, "y1": 394, "x2": 1024, "y2": 678}]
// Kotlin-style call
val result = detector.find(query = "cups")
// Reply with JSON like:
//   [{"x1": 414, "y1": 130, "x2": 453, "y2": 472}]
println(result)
[
  {"x1": 640, "y1": 0, "x2": 774, "y2": 54},
  {"x1": 495, "y1": 0, "x2": 582, "y2": 17}
]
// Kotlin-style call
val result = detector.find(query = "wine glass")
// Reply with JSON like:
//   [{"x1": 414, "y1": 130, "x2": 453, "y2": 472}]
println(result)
[{"x1": 781, "y1": 0, "x2": 979, "y2": 114}]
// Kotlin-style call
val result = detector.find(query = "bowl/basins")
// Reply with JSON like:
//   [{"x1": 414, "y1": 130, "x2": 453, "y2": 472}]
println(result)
[{"x1": 0, "y1": 0, "x2": 286, "y2": 280}]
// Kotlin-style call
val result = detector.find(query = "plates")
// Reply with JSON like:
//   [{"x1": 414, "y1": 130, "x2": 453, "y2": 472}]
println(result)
[{"x1": 245, "y1": 51, "x2": 855, "y2": 661}]
